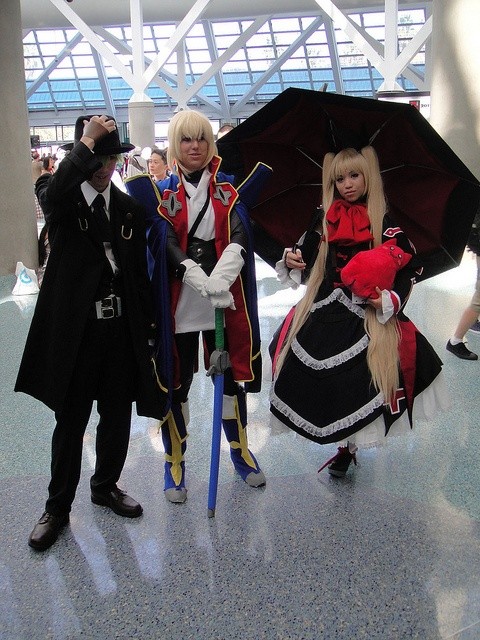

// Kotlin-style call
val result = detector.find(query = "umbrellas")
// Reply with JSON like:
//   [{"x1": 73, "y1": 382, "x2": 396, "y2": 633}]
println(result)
[{"x1": 218, "y1": 86, "x2": 479, "y2": 287}]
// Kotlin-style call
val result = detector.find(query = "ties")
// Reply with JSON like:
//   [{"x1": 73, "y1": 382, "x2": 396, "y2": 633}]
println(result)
[{"x1": 92, "y1": 194, "x2": 108, "y2": 234}]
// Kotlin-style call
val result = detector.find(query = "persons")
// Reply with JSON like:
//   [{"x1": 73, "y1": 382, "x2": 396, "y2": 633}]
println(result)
[
  {"x1": 163, "y1": 146, "x2": 177, "y2": 175},
  {"x1": 14, "y1": 114, "x2": 156, "y2": 551},
  {"x1": 126, "y1": 146, "x2": 147, "y2": 177},
  {"x1": 42, "y1": 156, "x2": 55, "y2": 177},
  {"x1": 216, "y1": 124, "x2": 234, "y2": 141},
  {"x1": 265, "y1": 144, "x2": 444, "y2": 476},
  {"x1": 34, "y1": 224, "x2": 53, "y2": 289},
  {"x1": 125, "y1": 110, "x2": 274, "y2": 504},
  {"x1": 148, "y1": 148, "x2": 170, "y2": 186},
  {"x1": 446, "y1": 214, "x2": 480, "y2": 361},
  {"x1": 31, "y1": 152, "x2": 42, "y2": 181}
]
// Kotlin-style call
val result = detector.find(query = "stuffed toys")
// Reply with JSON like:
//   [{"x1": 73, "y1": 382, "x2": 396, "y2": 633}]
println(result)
[{"x1": 340, "y1": 237, "x2": 413, "y2": 301}]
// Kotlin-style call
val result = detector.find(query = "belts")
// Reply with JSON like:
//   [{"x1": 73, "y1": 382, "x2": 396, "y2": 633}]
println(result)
[{"x1": 95, "y1": 294, "x2": 123, "y2": 320}]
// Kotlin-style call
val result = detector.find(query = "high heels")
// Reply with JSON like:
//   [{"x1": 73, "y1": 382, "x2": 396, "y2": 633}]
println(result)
[{"x1": 328, "y1": 441, "x2": 357, "y2": 477}]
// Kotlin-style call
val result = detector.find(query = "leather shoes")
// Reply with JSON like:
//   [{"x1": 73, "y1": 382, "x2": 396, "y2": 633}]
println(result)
[
  {"x1": 28, "y1": 511, "x2": 69, "y2": 551},
  {"x1": 91, "y1": 488, "x2": 143, "y2": 518},
  {"x1": 468, "y1": 320, "x2": 480, "y2": 335},
  {"x1": 446, "y1": 339, "x2": 478, "y2": 360}
]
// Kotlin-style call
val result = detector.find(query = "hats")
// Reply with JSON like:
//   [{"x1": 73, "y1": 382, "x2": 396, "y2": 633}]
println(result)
[
  {"x1": 59, "y1": 115, "x2": 135, "y2": 154},
  {"x1": 134, "y1": 146, "x2": 141, "y2": 155}
]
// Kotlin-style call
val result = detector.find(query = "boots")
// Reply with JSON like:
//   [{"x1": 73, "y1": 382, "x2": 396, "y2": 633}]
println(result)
[
  {"x1": 222, "y1": 393, "x2": 265, "y2": 487},
  {"x1": 161, "y1": 398, "x2": 190, "y2": 503}
]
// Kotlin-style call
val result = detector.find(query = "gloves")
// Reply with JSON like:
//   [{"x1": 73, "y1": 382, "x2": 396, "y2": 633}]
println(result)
[
  {"x1": 200, "y1": 243, "x2": 247, "y2": 297},
  {"x1": 176, "y1": 259, "x2": 236, "y2": 310}
]
